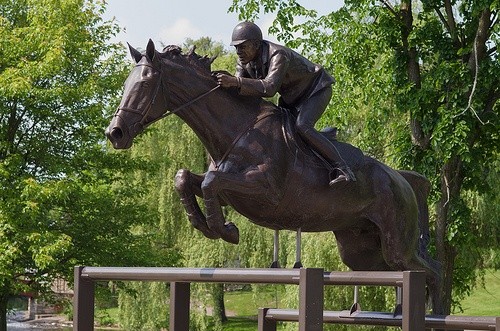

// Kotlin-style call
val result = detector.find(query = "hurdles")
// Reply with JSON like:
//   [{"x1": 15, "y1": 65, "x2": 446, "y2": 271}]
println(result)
[{"x1": 70, "y1": 262, "x2": 500, "y2": 331}]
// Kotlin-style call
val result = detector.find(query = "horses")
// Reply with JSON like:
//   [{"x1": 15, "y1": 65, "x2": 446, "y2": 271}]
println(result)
[{"x1": 105, "y1": 38, "x2": 445, "y2": 317}]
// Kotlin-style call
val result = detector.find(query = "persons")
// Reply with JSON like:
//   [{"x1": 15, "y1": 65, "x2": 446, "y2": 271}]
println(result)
[{"x1": 216, "y1": 21, "x2": 357, "y2": 189}]
